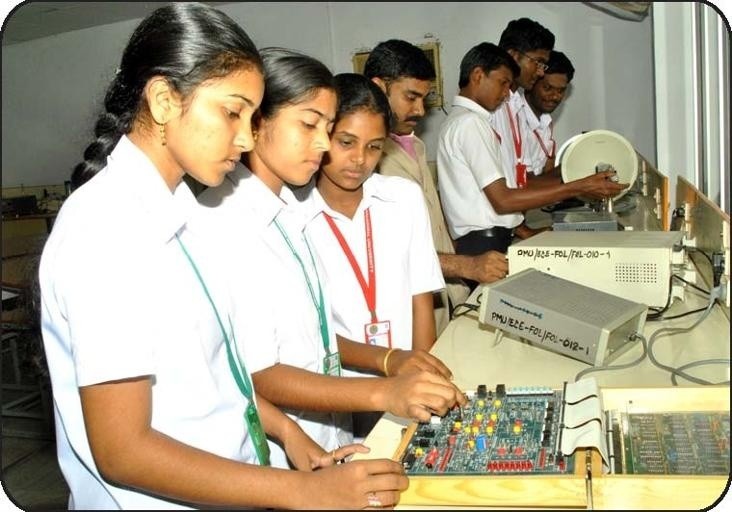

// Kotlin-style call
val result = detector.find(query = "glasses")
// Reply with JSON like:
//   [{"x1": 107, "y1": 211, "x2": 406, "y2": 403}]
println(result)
[{"x1": 520, "y1": 51, "x2": 549, "y2": 71}]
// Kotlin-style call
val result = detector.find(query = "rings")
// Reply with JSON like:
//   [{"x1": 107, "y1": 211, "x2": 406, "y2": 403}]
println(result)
[{"x1": 368, "y1": 492, "x2": 383, "y2": 506}]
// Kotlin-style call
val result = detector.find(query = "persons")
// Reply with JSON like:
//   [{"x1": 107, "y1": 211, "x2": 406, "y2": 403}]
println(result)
[
  {"x1": 363, "y1": 39, "x2": 509, "y2": 339},
  {"x1": 436, "y1": 18, "x2": 630, "y2": 285},
  {"x1": 192, "y1": 46, "x2": 469, "y2": 453},
  {"x1": 39, "y1": 3, "x2": 408, "y2": 512},
  {"x1": 289, "y1": 73, "x2": 452, "y2": 382}
]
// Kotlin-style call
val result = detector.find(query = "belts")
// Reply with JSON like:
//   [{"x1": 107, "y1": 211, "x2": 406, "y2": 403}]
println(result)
[{"x1": 470, "y1": 227, "x2": 511, "y2": 237}]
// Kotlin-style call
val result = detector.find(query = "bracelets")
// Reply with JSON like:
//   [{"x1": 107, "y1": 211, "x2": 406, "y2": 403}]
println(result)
[{"x1": 383, "y1": 347, "x2": 402, "y2": 377}]
